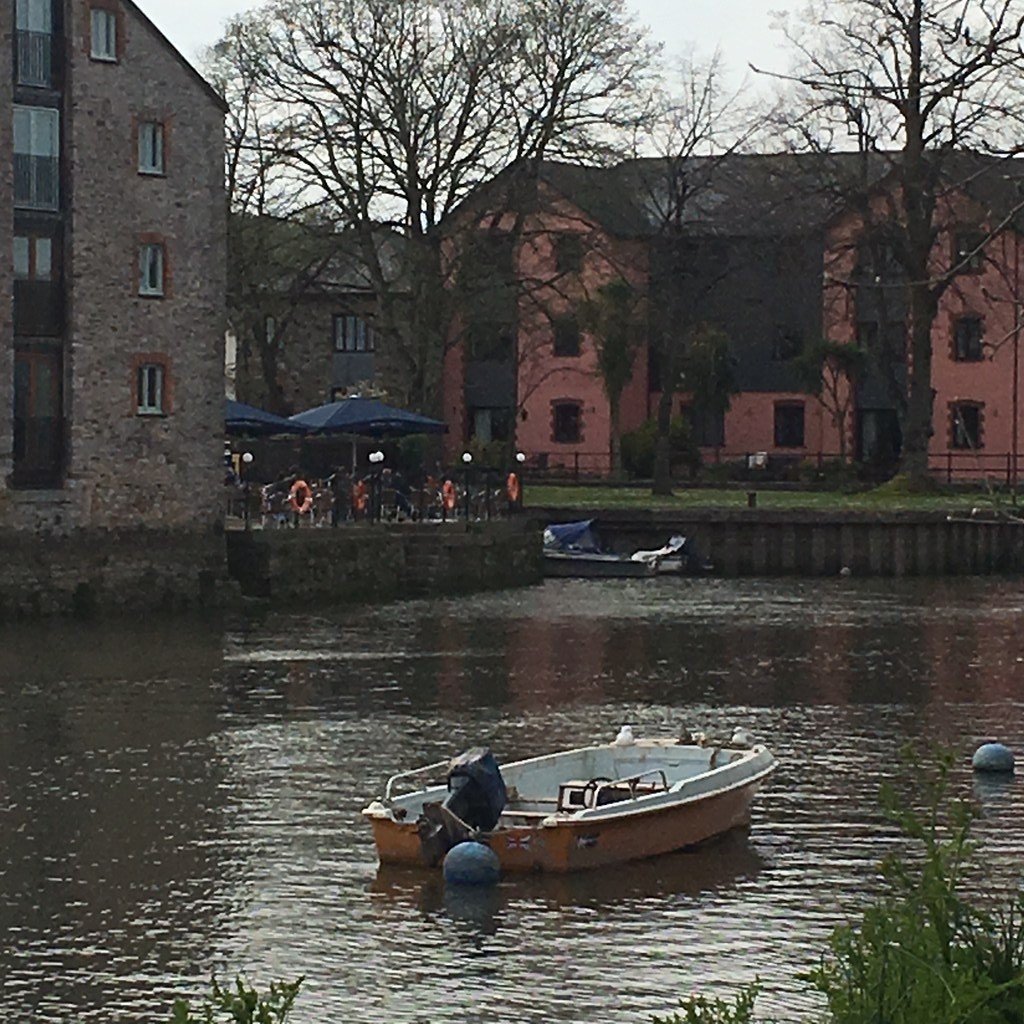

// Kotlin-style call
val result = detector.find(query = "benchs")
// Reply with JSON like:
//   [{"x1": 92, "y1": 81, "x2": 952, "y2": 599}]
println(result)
[
  {"x1": 508, "y1": 797, "x2": 558, "y2": 804},
  {"x1": 502, "y1": 811, "x2": 551, "y2": 826}
]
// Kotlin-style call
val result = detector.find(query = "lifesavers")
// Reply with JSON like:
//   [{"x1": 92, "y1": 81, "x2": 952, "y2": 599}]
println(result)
[
  {"x1": 507, "y1": 472, "x2": 519, "y2": 501},
  {"x1": 442, "y1": 480, "x2": 455, "y2": 510},
  {"x1": 290, "y1": 480, "x2": 312, "y2": 513}
]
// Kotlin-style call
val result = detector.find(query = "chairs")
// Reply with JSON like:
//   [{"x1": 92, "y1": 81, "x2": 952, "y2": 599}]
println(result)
[{"x1": 260, "y1": 489, "x2": 508, "y2": 528}]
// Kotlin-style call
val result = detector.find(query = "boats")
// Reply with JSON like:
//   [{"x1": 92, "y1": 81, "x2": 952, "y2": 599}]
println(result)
[
  {"x1": 361, "y1": 724, "x2": 779, "y2": 875},
  {"x1": 541, "y1": 516, "x2": 692, "y2": 577}
]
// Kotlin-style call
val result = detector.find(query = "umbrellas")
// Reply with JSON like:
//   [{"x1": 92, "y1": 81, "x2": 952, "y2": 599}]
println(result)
[
  {"x1": 284, "y1": 397, "x2": 449, "y2": 483},
  {"x1": 226, "y1": 394, "x2": 306, "y2": 480}
]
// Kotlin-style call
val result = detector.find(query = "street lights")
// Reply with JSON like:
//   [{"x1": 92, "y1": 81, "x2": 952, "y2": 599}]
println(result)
[
  {"x1": 368, "y1": 450, "x2": 385, "y2": 525},
  {"x1": 462, "y1": 451, "x2": 472, "y2": 533},
  {"x1": 242, "y1": 452, "x2": 254, "y2": 531},
  {"x1": 516, "y1": 453, "x2": 525, "y2": 512}
]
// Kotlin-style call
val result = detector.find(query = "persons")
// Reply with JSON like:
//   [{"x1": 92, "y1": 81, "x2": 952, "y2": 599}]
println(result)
[{"x1": 266, "y1": 459, "x2": 463, "y2": 519}]
